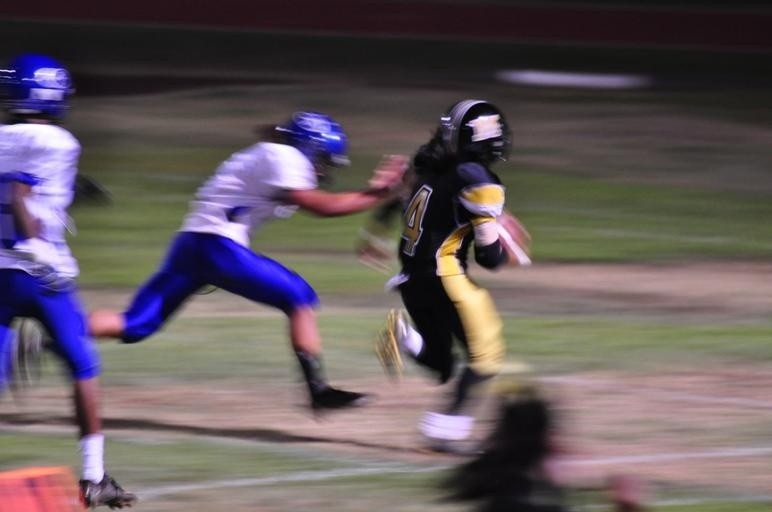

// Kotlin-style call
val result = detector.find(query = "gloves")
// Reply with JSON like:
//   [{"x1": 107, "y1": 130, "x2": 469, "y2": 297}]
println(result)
[
  {"x1": 497, "y1": 213, "x2": 531, "y2": 266},
  {"x1": 368, "y1": 155, "x2": 409, "y2": 191},
  {"x1": 16, "y1": 239, "x2": 78, "y2": 292}
]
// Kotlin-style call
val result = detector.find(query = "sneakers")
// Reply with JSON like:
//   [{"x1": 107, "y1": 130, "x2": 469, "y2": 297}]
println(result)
[
  {"x1": 374, "y1": 308, "x2": 407, "y2": 374},
  {"x1": 311, "y1": 388, "x2": 363, "y2": 409},
  {"x1": 78, "y1": 473, "x2": 136, "y2": 510}
]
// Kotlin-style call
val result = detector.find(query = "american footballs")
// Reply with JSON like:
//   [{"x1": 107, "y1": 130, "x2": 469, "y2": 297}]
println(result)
[{"x1": 497, "y1": 208, "x2": 534, "y2": 267}]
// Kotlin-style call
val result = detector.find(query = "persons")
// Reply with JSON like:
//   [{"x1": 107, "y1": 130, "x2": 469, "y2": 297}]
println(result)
[
  {"x1": 8, "y1": 112, "x2": 407, "y2": 413},
  {"x1": 437, "y1": 386, "x2": 658, "y2": 511},
  {"x1": 0, "y1": 55, "x2": 137, "y2": 512},
  {"x1": 357, "y1": 95, "x2": 529, "y2": 453}
]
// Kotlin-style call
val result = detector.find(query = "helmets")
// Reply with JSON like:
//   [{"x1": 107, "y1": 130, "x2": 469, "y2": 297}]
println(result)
[
  {"x1": 439, "y1": 99, "x2": 509, "y2": 161},
  {"x1": 0, "y1": 53, "x2": 77, "y2": 114},
  {"x1": 276, "y1": 111, "x2": 350, "y2": 165}
]
